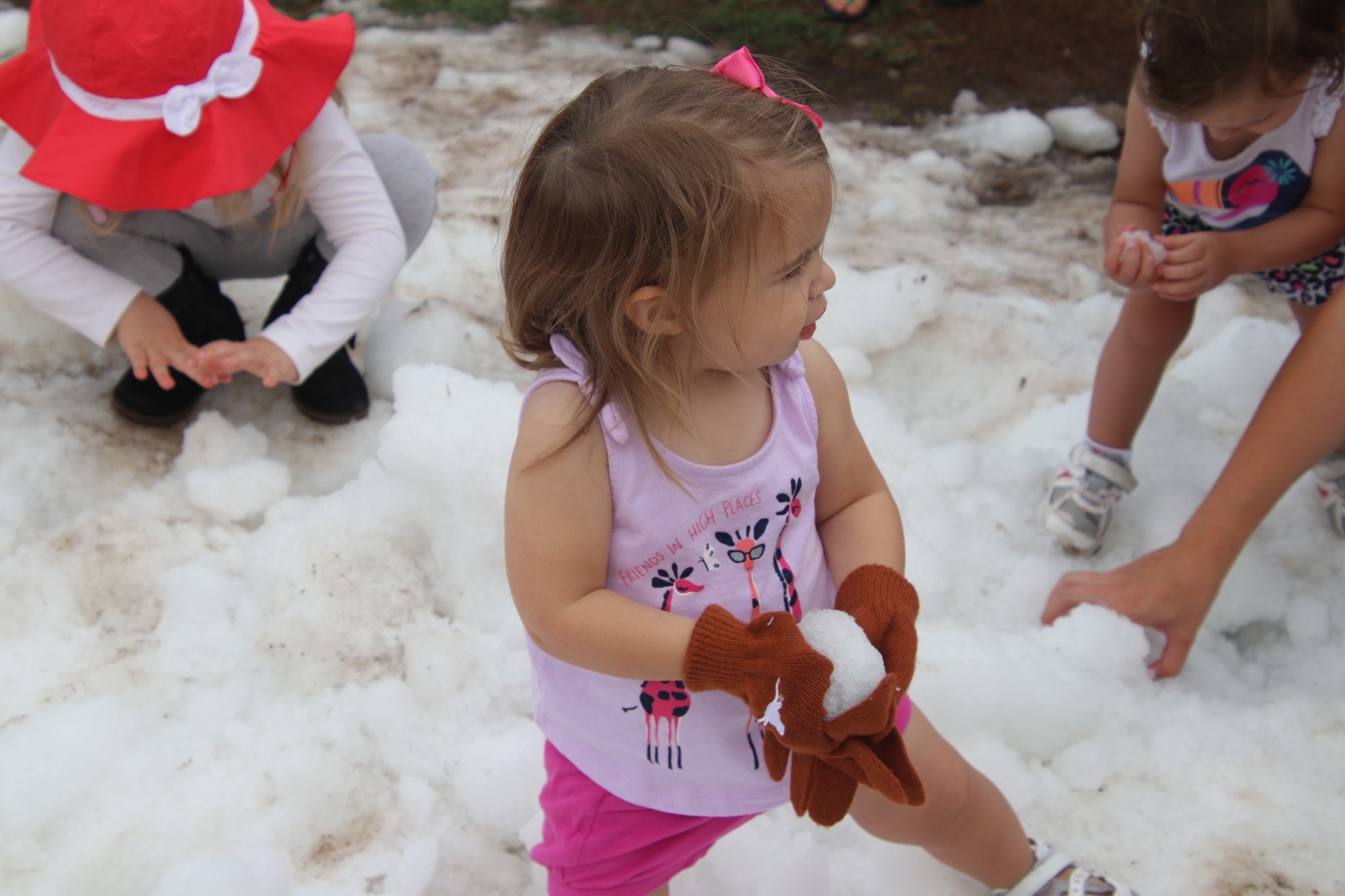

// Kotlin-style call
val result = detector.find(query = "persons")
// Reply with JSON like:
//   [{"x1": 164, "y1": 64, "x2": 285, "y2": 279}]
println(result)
[
  {"x1": 1041, "y1": 280, "x2": 1345, "y2": 675},
  {"x1": 1047, "y1": 0, "x2": 1345, "y2": 560},
  {"x1": 501, "y1": 68, "x2": 1134, "y2": 896},
  {"x1": 0, "y1": 0, "x2": 440, "y2": 433}
]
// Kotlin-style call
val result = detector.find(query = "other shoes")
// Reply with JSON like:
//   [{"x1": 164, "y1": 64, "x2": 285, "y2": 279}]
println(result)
[{"x1": 989, "y1": 835, "x2": 1135, "y2": 896}]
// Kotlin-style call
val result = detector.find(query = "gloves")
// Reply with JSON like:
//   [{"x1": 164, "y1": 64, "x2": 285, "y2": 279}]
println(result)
[{"x1": 682, "y1": 561, "x2": 930, "y2": 827}]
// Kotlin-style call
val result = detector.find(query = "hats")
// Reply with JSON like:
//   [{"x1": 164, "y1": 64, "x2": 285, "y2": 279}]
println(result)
[{"x1": 0, "y1": 2, "x2": 355, "y2": 214}]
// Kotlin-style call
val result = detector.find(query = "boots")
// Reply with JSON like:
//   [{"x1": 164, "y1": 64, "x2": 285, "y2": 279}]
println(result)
[
  {"x1": 258, "y1": 237, "x2": 371, "y2": 426},
  {"x1": 109, "y1": 242, "x2": 247, "y2": 426}
]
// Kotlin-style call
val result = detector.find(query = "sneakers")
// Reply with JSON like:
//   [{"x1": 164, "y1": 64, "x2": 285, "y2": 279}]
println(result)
[
  {"x1": 1311, "y1": 450, "x2": 1345, "y2": 543},
  {"x1": 1045, "y1": 438, "x2": 1138, "y2": 559}
]
